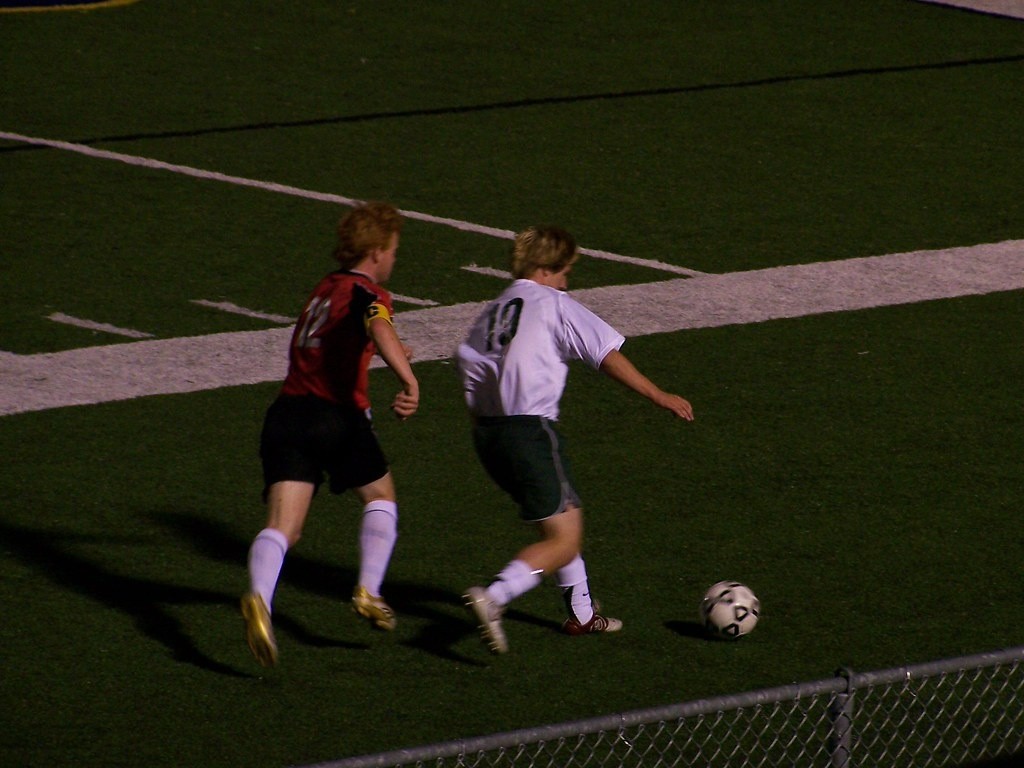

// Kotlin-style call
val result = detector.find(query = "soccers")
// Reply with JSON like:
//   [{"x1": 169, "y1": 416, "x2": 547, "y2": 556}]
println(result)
[{"x1": 699, "y1": 578, "x2": 762, "y2": 642}]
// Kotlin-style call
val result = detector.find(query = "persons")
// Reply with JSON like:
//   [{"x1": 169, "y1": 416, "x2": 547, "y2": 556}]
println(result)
[
  {"x1": 457, "y1": 226, "x2": 693, "y2": 653},
  {"x1": 242, "y1": 201, "x2": 419, "y2": 667}
]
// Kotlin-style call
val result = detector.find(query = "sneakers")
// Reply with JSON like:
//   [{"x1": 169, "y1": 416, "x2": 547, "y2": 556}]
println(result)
[
  {"x1": 465, "y1": 587, "x2": 510, "y2": 655},
  {"x1": 349, "y1": 585, "x2": 398, "y2": 631},
  {"x1": 240, "y1": 593, "x2": 279, "y2": 668},
  {"x1": 562, "y1": 615, "x2": 622, "y2": 635}
]
